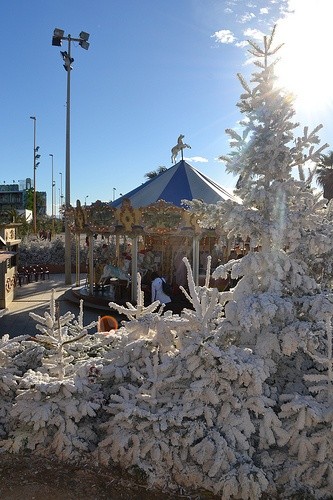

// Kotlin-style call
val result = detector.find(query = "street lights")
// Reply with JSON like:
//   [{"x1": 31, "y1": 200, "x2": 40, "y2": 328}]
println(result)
[
  {"x1": 85, "y1": 196, "x2": 88, "y2": 206},
  {"x1": 51, "y1": 27, "x2": 89, "y2": 287},
  {"x1": 49, "y1": 154, "x2": 55, "y2": 231},
  {"x1": 58, "y1": 173, "x2": 63, "y2": 222},
  {"x1": 29, "y1": 116, "x2": 42, "y2": 234}
]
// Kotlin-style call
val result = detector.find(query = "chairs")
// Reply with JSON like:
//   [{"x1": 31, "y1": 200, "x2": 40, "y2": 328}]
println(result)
[{"x1": 18, "y1": 265, "x2": 49, "y2": 285}]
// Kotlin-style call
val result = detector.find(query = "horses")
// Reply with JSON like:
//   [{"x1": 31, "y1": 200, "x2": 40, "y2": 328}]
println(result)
[{"x1": 96, "y1": 249, "x2": 158, "y2": 289}]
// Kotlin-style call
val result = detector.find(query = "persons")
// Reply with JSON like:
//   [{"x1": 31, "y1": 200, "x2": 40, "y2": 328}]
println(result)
[
  {"x1": 97, "y1": 315, "x2": 118, "y2": 333},
  {"x1": 150, "y1": 271, "x2": 173, "y2": 315},
  {"x1": 38, "y1": 225, "x2": 112, "y2": 272}
]
